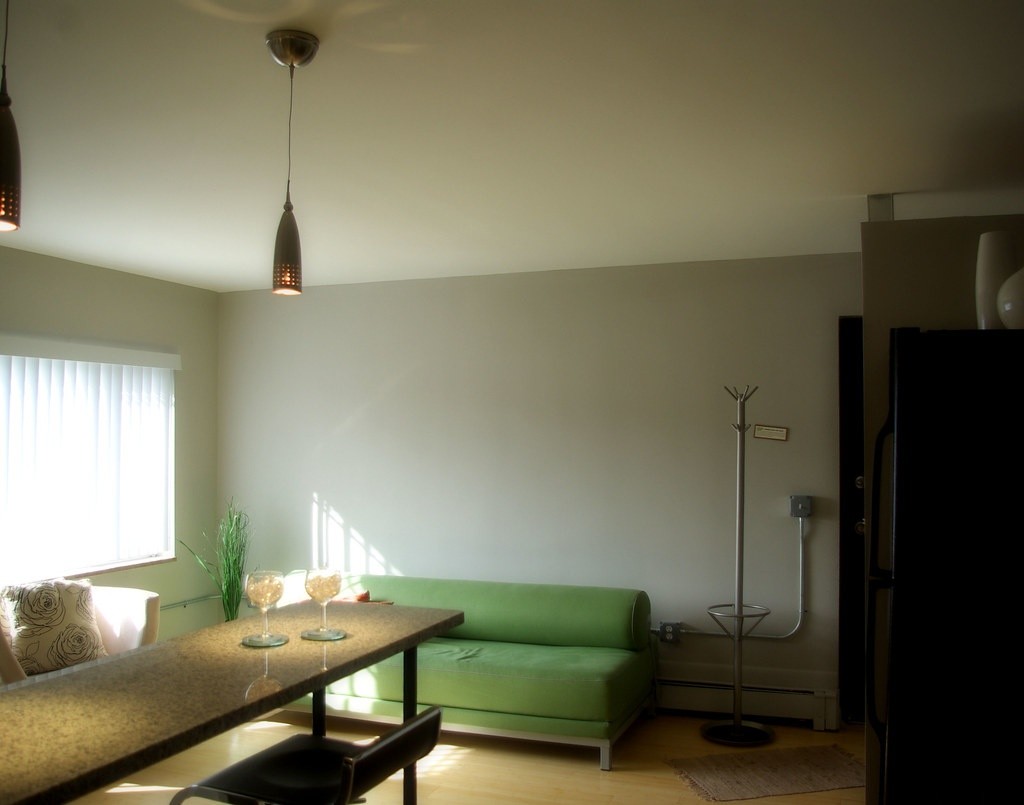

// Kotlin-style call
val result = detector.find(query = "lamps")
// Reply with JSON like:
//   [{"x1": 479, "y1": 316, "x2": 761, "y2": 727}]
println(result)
[
  {"x1": 265, "y1": 30, "x2": 321, "y2": 297},
  {"x1": 0, "y1": 0, "x2": 19, "y2": 233}
]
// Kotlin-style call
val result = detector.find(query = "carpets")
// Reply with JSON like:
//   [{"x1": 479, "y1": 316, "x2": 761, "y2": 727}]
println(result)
[{"x1": 667, "y1": 742, "x2": 868, "y2": 803}]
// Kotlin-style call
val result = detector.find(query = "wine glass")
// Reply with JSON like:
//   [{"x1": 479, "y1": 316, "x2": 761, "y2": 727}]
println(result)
[
  {"x1": 305, "y1": 569, "x2": 342, "y2": 635},
  {"x1": 245, "y1": 572, "x2": 284, "y2": 641}
]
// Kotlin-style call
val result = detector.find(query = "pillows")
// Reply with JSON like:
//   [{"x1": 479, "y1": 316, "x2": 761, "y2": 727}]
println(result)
[{"x1": 0, "y1": 578, "x2": 107, "y2": 676}]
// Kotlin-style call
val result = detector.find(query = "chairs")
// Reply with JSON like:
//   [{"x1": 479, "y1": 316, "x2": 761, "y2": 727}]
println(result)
[{"x1": 168, "y1": 704, "x2": 441, "y2": 805}]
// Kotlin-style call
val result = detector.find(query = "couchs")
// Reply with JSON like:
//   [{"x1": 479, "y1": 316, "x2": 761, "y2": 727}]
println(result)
[{"x1": 259, "y1": 568, "x2": 655, "y2": 771}]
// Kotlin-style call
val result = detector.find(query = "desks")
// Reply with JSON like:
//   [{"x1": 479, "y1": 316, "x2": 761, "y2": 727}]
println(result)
[{"x1": 0, "y1": 596, "x2": 463, "y2": 805}]
